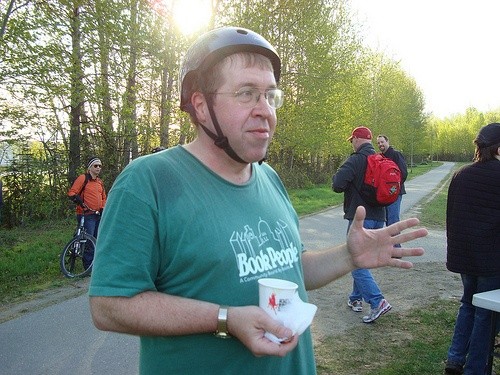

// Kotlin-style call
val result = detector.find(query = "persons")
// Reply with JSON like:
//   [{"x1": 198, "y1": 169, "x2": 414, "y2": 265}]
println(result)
[
  {"x1": 377, "y1": 135, "x2": 408, "y2": 258},
  {"x1": 88, "y1": 27, "x2": 428, "y2": 375},
  {"x1": 445, "y1": 123, "x2": 500, "y2": 375},
  {"x1": 67, "y1": 157, "x2": 106, "y2": 276},
  {"x1": 331, "y1": 126, "x2": 393, "y2": 322}
]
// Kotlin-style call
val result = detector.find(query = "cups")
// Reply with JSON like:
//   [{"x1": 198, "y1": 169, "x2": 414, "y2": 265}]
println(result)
[{"x1": 258, "y1": 278, "x2": 299, "y2": 317}]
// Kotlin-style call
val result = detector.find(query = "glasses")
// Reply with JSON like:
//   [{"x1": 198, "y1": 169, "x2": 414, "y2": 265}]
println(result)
[
  {"x1": 93, "y1": 165, "x2": 101, "y2": 168},
  {"x1": 209, "y1": 85, "x2": 286, "y2": 109}
]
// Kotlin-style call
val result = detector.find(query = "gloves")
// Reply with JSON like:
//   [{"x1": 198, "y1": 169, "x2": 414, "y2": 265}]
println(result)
[
  {"x1": 73, "y1": 195, "x2": 82, "y2": 207},
  {"x1": 99, "y1": 208, "x2": 103, "y2": 215}
]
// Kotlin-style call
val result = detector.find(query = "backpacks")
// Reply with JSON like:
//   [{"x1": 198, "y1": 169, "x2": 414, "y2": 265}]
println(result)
[{"x1": 350, "y1": 149, "x2": 402, "y2": 207}]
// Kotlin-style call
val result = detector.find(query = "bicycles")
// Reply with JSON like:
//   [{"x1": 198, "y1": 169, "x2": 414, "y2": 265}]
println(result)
[{"x1": 61, "y1": 205, "x2": 100, "y2": 277}]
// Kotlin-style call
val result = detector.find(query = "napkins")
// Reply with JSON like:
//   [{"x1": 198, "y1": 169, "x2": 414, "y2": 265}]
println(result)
[{"x1": 264, "y1": 292, "x2": 318, "y2": 345}]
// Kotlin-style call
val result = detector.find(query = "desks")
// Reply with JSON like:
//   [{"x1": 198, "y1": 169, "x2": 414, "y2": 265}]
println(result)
[{"x1": 471, "y1": 289, "x2": 500, "y2": 375}]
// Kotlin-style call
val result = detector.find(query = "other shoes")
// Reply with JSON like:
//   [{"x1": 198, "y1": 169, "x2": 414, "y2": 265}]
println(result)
[{"x1": 445, "y1": 364, "x2": 465, "y2": 375}]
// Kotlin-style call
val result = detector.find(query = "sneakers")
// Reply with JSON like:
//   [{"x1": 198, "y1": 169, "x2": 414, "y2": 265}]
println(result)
[
  {"x1": 363, "y1": 299, "x2": 392, "y2": 323},
  {"x1": 348, "y1": 298, "x2": 363, "y2": 312}
]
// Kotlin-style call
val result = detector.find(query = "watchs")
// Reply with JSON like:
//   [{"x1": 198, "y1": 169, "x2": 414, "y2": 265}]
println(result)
[{"x1": 213, "y1": 305, "x2": 232, "y2": 338}]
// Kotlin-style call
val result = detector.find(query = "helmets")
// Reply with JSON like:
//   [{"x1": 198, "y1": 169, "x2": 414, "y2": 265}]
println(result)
[{"x1": 176, "y1": 26, "x2": 281, "y2": 114}]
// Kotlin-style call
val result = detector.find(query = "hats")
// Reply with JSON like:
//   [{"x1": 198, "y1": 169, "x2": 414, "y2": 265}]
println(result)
[
  {"x1": 85, "y1": 156, "x2": 102, "y2": 170},
  {"x1": 347, "y1": 127, "x2": 371, "y2": 141}
]
